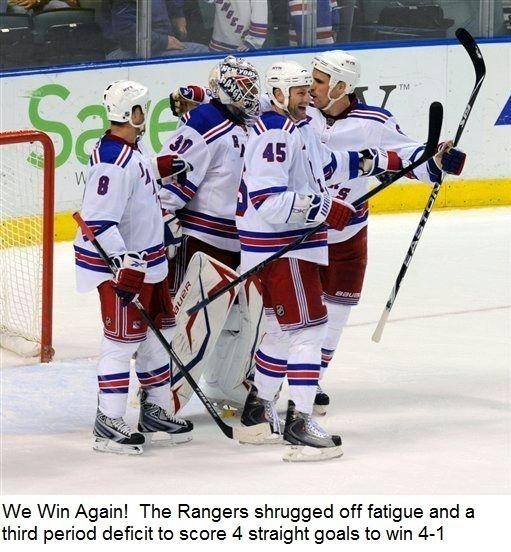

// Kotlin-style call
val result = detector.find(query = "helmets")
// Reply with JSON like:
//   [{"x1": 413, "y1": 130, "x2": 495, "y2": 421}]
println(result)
[
  {"x1": 265, "y1": 60, "x2": 314, "y2": 111},
  {"x1": 209, "y1": 49, "x2": 262, "y2": 128},
  {"x1": 311, "y1": 50, "x2": 358, "y2": 96},
  {"x1": 104, "y1": 80, "x2": 148, "y2": 129}
]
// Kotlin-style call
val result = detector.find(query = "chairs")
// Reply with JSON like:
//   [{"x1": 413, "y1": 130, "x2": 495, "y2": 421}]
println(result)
[{"x1": 0, "y1": 0, "x2": 454, "y2": 75}]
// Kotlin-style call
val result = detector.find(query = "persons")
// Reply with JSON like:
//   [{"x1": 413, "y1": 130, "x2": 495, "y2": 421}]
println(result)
[
  {"x1": 73, "y1": 79, "x2": 192, "y2": 443},
  {"x1": 235, "y1": 61, "x2": 407, "y2": 448},
  {"x1": 155, "y1": 53, "x2": 262, "y2": 414},
  {"x1": 168, "y1": 50, "x2": 467, "y2": 408},
  {"x1": 10, "y1": 0, "x2": 338, "y2": 62}
]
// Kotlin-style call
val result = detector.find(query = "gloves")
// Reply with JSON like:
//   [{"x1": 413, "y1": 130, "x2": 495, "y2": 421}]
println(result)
[
  {"x1": 151, "y1": 152, "x2": 187, "y2": 181},
  {"x1": 361, "y1": 145, "x2": 414, "y2": 177},
  {"x1": 304, "y1": 192, "x2": 355, "y2": 231},
  {"x1": 112, "y1": 253, "x2": 147, "y2": 307}
]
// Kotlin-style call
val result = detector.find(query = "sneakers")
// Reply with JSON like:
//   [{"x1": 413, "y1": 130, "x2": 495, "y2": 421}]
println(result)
[
  {"x1": 316, "y1": 385, "x2": 330, "y2": 405},
  {"x1": 283, "y1": 414, "x2": 341, "y2": 446},
  {"x1": 137, "y1": 402, "x2": 194, "y2": 433},
  {"x1": 241, "y1": 394, "x2": 285, "y2": 435},
  {"x1": 93, "y1": 407, "x2": 146, "y2": 445}
]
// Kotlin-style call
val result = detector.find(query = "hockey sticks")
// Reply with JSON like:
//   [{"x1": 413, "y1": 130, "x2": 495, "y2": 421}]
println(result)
[{"x1": 73, "y1": 213, "x2": 275, "y2": 440}]
